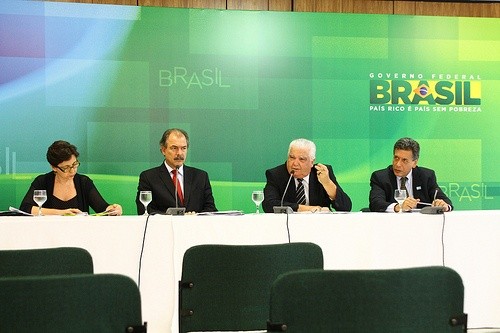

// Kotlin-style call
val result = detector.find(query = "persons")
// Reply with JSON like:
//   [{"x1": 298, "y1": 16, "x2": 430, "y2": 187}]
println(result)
[
  {"x1": 369, "y1": 138, "x2": 453, "y2": 213},
  {"x1": 262, "y1": 139, "x2": 352, "y2": 213},
  {"x1": 136, "y1": 128, "x2": 218, "y2": 216},
  {"x1": 19, "y1": 140, "x2": 122, "y2": 216}
]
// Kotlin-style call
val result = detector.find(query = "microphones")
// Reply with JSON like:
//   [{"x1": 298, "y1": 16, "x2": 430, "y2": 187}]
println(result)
[
  {"x1": 166, "y1": 165, "x2": 186, "y2": 216},
  {"x1": 272, "y1": 170, "x2": 295, "y2": 214},
  {"x1": 420, "y1": 188, "x2": 445, "y2": 214}
]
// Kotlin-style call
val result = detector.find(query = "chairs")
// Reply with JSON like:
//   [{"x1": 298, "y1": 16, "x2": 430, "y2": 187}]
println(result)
[
  {"x1": 0, "y1": 273, "x2": 148, "y2": 333},
  {"x1": 178, "y1": 243, "x2": 324, "y2": 333},
  {"x1": 0, "y1": 247, "x2": 94, "y2": 278},
  {"x1": 268, "y1": 265, "x2": 467, "y2": 333}
]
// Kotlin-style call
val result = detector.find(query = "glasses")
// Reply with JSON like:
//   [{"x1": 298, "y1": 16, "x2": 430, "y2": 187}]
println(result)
[{"x1": 56, "y1": 160, "x2": 80, "y2": 173}]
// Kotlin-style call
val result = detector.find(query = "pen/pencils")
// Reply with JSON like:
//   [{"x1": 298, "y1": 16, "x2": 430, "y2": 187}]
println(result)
[{"x1": 417, "y1": 202, "x2": 432, "y2": 205}]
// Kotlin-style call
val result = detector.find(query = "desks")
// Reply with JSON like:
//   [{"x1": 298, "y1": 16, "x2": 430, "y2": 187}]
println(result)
[{"x1": 1, "y1": 210, "x2": 499, "y2": 333}]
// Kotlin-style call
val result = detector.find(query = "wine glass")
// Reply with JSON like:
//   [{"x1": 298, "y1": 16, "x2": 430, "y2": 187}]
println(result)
[
  {"x1": 394, "y1": 190, "x2": 407, "y2": 214},
  {"x1": 252, "y1": 191, "x2": 264, "y2": 214},
  {"x1": 33, "y1": 190, "x2": 47, "y2": 216},
  {"x1": 139, "y1": 190, "x2": 152, "y2": 216}
]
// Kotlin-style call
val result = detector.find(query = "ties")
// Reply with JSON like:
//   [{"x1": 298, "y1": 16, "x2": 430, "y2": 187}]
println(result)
[
  {"x1": 295, "y1": 178, "x2": 306, "y2": 205},
  {"x1": 171, "y1": 170, "x2": 184, "y2": 206},
  {"x1": 400, "y1": 177, "x2": 409, "y2": 198}
]
